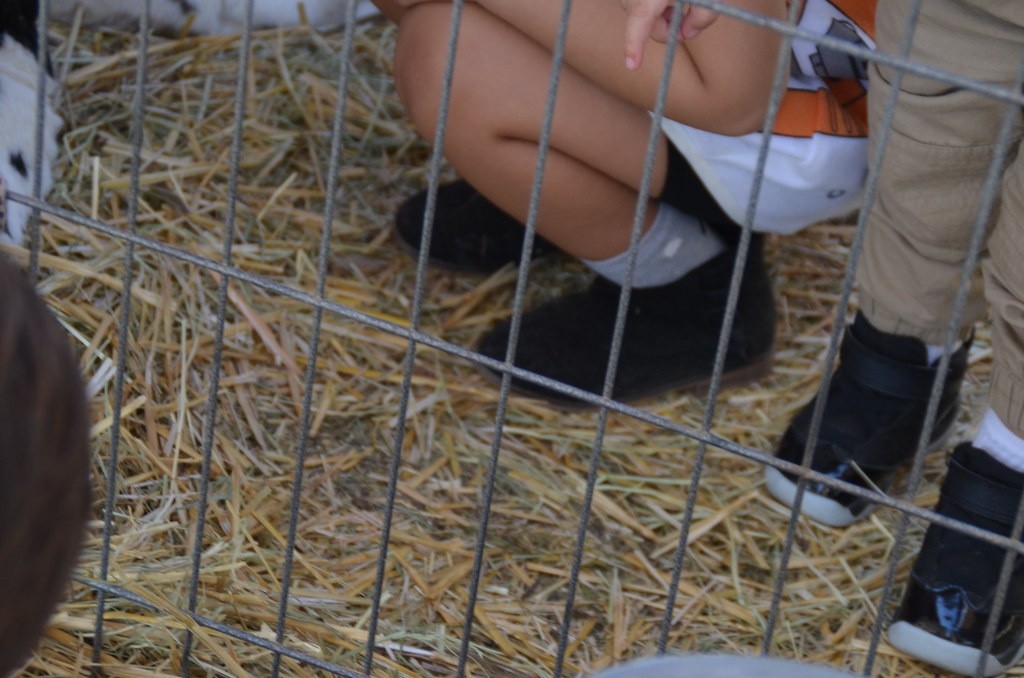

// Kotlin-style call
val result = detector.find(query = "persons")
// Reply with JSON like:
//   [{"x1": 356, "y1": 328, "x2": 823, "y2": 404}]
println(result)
[
  {"x1": 765, "y1": 1, "x2": 1022, "y2": 677},
  {"x1": 364, "y1": 0, "x2": 877, "y2": 410}
]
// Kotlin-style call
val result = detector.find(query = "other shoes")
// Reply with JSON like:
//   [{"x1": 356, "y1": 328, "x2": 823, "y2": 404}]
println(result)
[
  {"x1": 885, "y1": 439, "x2": 1024, "y2": 675},
  {"x1": 765, "y1": 307, "x2": 975, "y2": 527},
  {"x1": 395, "y1": 142, "x2": 765, "y2": 268},
  {"x1": 474, "y1": 230, "x2": 774, "y2": 407}
]
